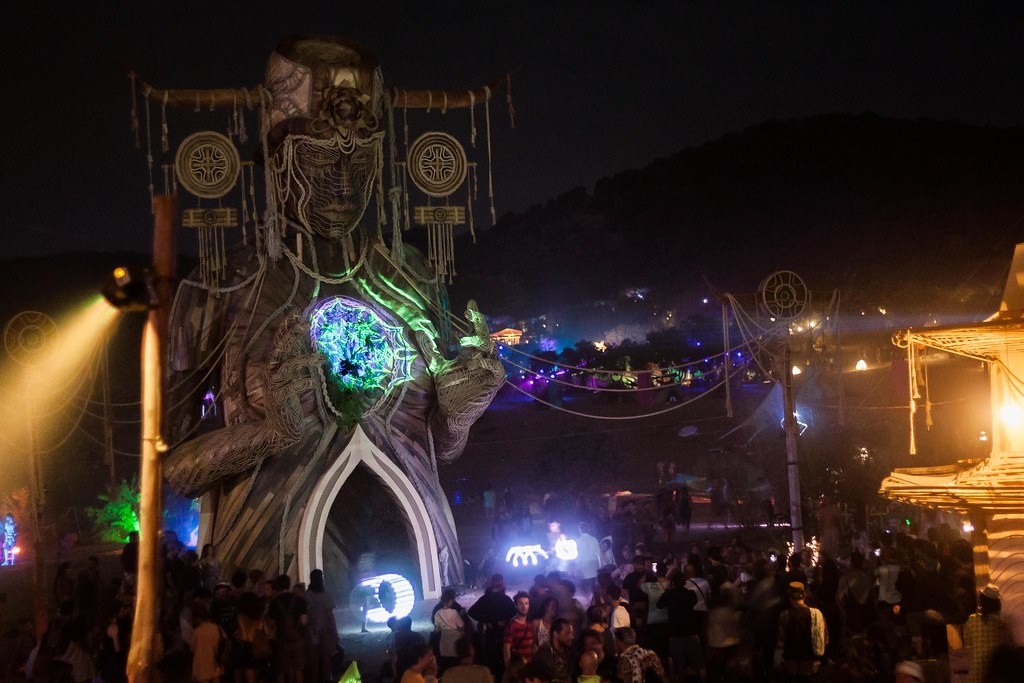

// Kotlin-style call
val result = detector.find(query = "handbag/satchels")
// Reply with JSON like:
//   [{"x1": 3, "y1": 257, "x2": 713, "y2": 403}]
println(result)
[{"x1": 217, "y1": 626, "x2": 233, "y2": 664}]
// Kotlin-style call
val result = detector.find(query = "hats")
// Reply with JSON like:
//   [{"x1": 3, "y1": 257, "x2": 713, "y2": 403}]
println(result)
[
  {"x1": 979, "y1": 583, "x2": 1001, "y2": 599},
  {"x1": 790, "y1": 581, "x2": 804, "y2": 593}
]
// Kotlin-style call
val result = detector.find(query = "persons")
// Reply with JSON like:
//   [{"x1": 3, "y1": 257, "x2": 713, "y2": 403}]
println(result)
[
  {"x1": 124, "y1": 27, "x2": 518, "y2": 599},
  {"x1": 1, "y1": 513, "x2": 18, "y2": 566},
  {"x1": 342, "y1": 472, "x2": 1024, "y2": 683},
  {"x1": 22, "y1": 530, "x2": 341, "y2": 683}
]
